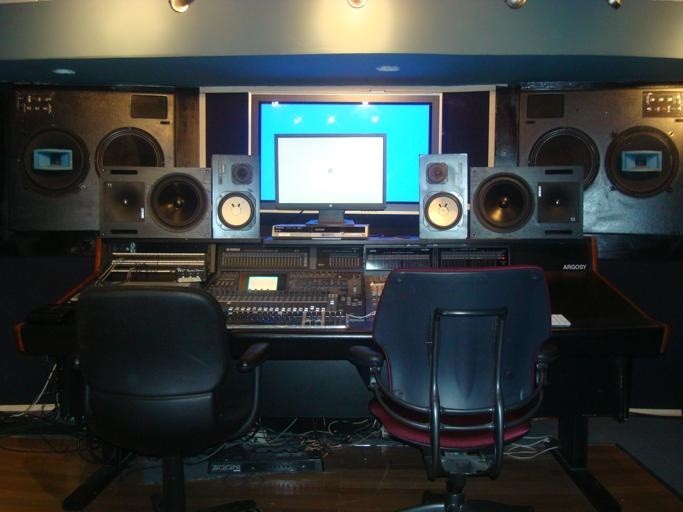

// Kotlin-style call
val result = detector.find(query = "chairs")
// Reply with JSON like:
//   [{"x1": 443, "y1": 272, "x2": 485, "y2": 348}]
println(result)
[
  {"x1": 41, "y1": 286, "x2": 267, "y2": 509},
  {"x1": 346, "y1": 265, "x2": 564, "y2": 510}
]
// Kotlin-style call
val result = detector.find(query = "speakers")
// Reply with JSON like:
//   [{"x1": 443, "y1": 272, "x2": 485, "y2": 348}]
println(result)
[
  {"x1": 1, "y1": 85, "x2": 175, "y2": 234},
  {"x1": 211, "y1": 152, "x2": 260, "y2": 241},
  {"x1": 417, "y1": 154, "x2": 469, "y2": 240},
  {"x1": 468, "y1": 166, "x2": 587, "y2": 240},
  {"x1": 517, "y1": 83, "x2": 683, "y2": 239},
  {"x1": 440, "y1": 85, "x2": 496, "y2": 168},
  {"x1": 198, "y1": 85, "x2": 248, "y2": 168},
  {"x1": 97, "y1": 165, "x2": 213, "y2": 240}
]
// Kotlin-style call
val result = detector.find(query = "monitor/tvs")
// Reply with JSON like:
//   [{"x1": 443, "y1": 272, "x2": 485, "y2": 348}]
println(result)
[
  {"x1": 246, "y1": 86, "x2": 444, "y2": 219},
  {"x1": 270, "y1": 133, "x2": 388, "y2": 223}
]
